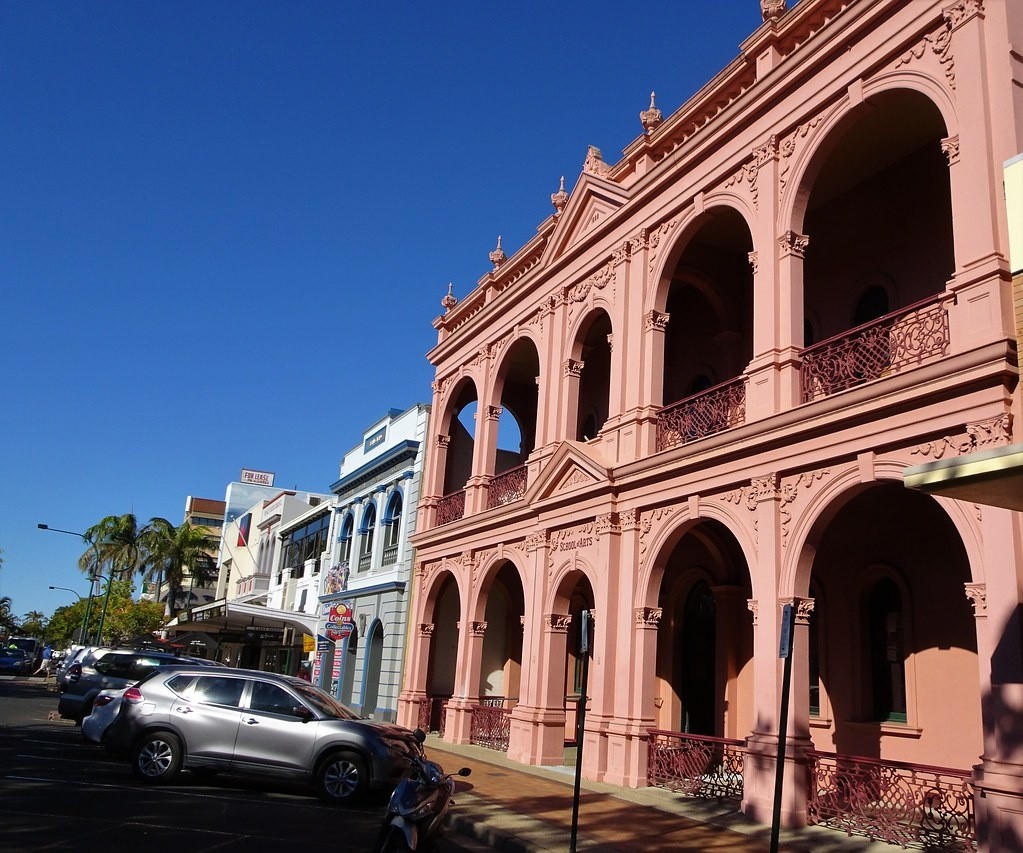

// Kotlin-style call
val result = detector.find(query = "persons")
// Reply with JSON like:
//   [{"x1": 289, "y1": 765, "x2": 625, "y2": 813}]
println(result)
[
  {"x1": 34, "y1": 645, "x2": 54, "y2": 680},
  {"x1": 8, "y1": 642, "x2": 18, "y2": 649}
]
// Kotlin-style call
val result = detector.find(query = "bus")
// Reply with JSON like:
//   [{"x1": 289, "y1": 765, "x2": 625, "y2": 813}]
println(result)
[{"x1": 7, "y1": 635, "x2": 42, "y2": 672}]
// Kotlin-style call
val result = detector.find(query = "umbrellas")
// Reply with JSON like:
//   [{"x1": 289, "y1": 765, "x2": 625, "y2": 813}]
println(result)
[{"x1": 121, "y1": 631, "x2": 220, "y2": 650}]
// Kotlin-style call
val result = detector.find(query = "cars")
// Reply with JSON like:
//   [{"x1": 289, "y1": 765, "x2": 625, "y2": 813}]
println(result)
[{"x1": 0, "y1": 645, "x2": 34, "y2": 677}]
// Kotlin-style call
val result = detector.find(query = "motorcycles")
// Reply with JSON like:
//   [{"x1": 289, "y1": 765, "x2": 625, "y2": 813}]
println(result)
[{"x1": 377, "y1": 728, "x2": 472, "y2": 853}]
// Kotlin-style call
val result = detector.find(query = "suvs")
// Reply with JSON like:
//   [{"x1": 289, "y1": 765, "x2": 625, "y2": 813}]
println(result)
[
  {"x1": 47, "y1": 643, "x2": 244, "y2": 745},
  {"x1": 106, "y1": 664, "x2": 427, "y2": 812}
]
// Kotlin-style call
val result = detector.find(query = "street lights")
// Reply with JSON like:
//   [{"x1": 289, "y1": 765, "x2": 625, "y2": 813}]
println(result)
[{"x1": 37, "y1": 523, "x2": 139, "y2": 646}]
